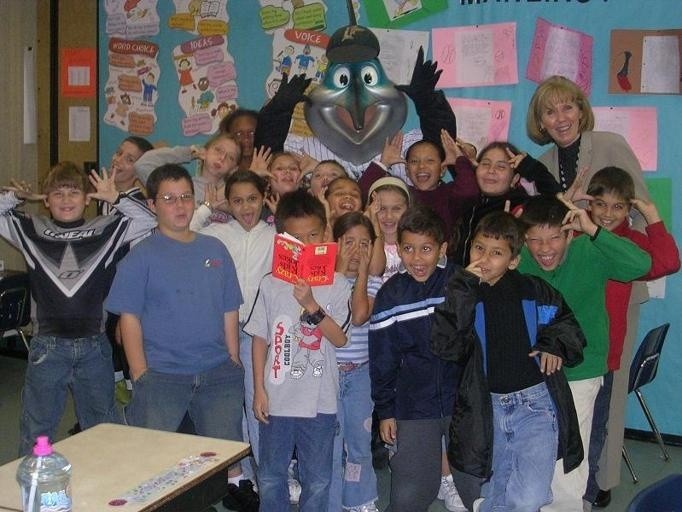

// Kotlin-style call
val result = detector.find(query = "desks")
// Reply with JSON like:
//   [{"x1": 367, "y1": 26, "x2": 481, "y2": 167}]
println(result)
[{"x1": 0, "y1": 422, "x2": 253, "y2": 512}]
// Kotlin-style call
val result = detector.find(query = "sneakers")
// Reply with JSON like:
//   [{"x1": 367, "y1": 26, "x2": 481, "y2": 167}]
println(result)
[
  {"x1": 346, "y1": 500, "x2": 382, "y2": 512},
  {"x1": 125, "y1": 377, "x2": 134, "y2": 390},
  {"x1": 222, "y1": 479, "x2": 261, "y2": 511},
  {"x1": 285, "y1": 460, "x2": 303, "y2": 505},
  {"x1": 114, "y1": 370, "x2": 124, "y2": 383},
  {"x1": 435, "y1": 472, "x2": 469, "y2": 511},
  {"x1": 472, "y1": 497, "x2": 485, "y2": 511}
]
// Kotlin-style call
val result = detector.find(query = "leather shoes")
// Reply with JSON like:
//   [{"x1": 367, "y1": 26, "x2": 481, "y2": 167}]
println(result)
[{"x1": 593, "y1": 487, "x2": 612, "y2": 508}]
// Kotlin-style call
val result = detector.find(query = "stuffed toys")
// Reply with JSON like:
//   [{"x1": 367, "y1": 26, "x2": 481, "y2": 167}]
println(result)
[{"x1": 253, "y1": 24, "x2": 457, "y2": 187}]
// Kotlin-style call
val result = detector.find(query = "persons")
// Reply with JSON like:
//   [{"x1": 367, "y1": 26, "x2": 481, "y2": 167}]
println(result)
[{"x1": 2, "y1": 74, "x2": 682, "y2": 512}]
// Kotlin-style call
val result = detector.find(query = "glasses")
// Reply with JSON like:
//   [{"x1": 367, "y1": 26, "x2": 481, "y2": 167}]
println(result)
[{"x1": 151, "y1": 192, "x2": 194, "y2": 204}]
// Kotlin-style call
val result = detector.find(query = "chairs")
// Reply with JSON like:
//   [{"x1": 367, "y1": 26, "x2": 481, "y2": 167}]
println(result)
[
  {"x1": 623, "y1": 324, "x2": 670, "y2": 484},
  {"x1": 625, "y1": 473, "x2": 681, "y2": 511},
  {"x1": 0, "y1": 268, "x2": 31, "y2": 361}
]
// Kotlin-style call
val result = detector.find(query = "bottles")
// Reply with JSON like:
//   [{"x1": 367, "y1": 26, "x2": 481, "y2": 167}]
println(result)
[{"x1": 16, "y1": 435, "x2": 73, "y2": 511}]
